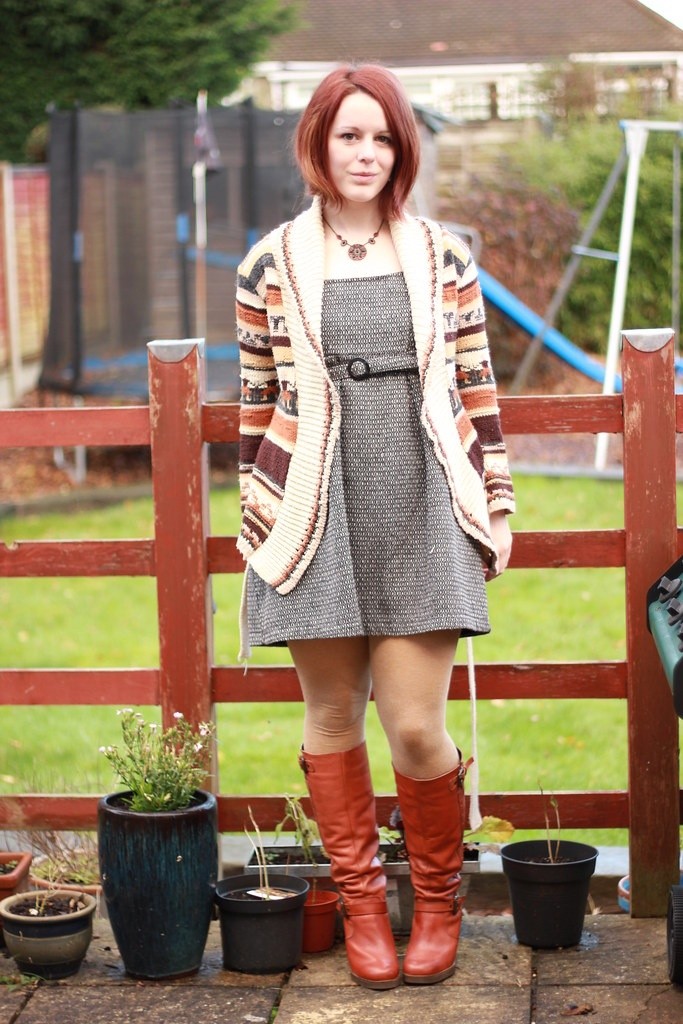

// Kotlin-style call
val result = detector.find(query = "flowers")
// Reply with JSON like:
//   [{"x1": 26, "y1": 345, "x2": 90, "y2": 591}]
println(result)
[{"x1": 98, "y1": 708, "x2": 219, "y2": 813}]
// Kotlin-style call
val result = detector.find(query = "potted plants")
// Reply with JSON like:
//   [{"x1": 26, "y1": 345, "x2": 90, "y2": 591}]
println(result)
[
  {"x1": 243, "y1": 793, "x2": 515, "y2": 937},
  {"x1": 500, "y1": 776, "x2": 599, "y2": 949}
]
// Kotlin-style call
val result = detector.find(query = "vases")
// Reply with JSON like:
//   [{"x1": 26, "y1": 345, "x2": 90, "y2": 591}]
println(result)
[
  {"x1": 29, "y1": 850, "x2": 102, "y2": 896},
  {"x1": 0, "y1": 891, "x2": 98, "y2": 979},
  {"x1": 97, "y1": 786, "x2": 219, "y2": 981},
  {"x1": 215, "y1": 875, "x2": 311, "y2": 974},
  {"x1": 302, "y1": 888, "x2": 340, "y2": 953},
  {"x1": 0, "y1": 851, "x2": 32, "y2": 947}
]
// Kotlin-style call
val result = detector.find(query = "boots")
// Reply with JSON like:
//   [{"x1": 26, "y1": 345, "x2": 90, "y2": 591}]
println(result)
[
  {"x1": 390, "y1": 748, "x2": 466, "y2": 986},
  {"x1": 297, "y1": 741, "x2": 401, "y2": 989}
]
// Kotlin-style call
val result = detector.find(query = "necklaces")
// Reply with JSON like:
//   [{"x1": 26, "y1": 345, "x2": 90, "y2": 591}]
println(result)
[{"x1": 323, "y1": 217, "x2": 389, "y2": 262}]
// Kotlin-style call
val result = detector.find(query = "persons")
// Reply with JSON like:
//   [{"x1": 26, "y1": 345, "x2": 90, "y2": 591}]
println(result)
[{"x1": 231, "y1": 69, "x2": 517, "y2": 985}]
